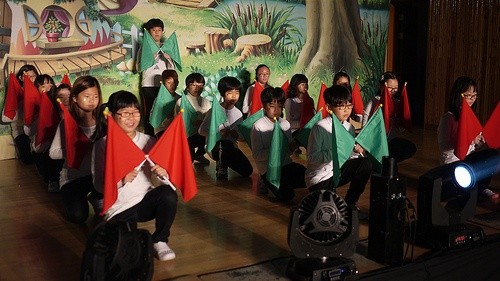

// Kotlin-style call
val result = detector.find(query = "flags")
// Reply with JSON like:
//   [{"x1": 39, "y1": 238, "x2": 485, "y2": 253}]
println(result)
[
  {"x1": 1, "y1": 72, "x2": 91, "y2": 170},
  {"x1": 453, "y1": 96, "x2": 500, "y2": 161},
  {"x1": 99, "y1": 109, "x2": 199, "y2": 217},
  {"x1": 135, "y1": 29, "x2": 183, "y2": 73},
  {"x1": 148, "y1": 79, "x2": 410, "y2": 192}
]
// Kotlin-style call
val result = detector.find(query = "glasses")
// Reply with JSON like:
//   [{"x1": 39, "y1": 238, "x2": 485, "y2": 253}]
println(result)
[
  {"x1": 386, "y1": 87, "x2": 399, "y2": 91},
  {"x1": 188, "y1": 83, "x2": 205, "y2": 88},
  {"x1": 335, "y1": 104, "x2": 355, "y2": 110},
  {"x1": 461, "y1": 94, "x2": 480, "y2": 98},
  {"x1": 117, "y1": 111, "x2": 141, "y2": 118}
]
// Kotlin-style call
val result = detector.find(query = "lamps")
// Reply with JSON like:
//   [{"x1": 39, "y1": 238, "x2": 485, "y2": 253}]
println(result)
[{"x1": 454, "y1": 150, "x2": 500, "y2": 190}]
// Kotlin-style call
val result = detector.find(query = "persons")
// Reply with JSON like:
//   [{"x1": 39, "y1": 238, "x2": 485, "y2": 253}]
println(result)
[
  {"x1": 437, "y1": 76, "x2": 500, "y2": 200},
  {"x1": 242, "y1": 64, "x2": 270, "y2": 117},
  {"x1": 10, "y1": 65, "x2": 39, "y2": 164},
  {"x1": 149, "y1": 69, "x2": 182, "y2": 140},
  {"x1": 136, "y1": 18, "x2": 175, "y2": 137},
  {"x1": 48, "y1": 75, "x2": 107, "y2": 224},
  {"x1": 198, "y1": 76, "x2": 253, "y2": 183},
  {"x1": 282, "y1": 74, "x2": 317, "y2": 136},
  {"x1": 174, "y1": 72, "x2": 212, "y2": 166},
  {"x1": 303, "y1": 84, "x2": 372, "y2": 216},
  {"x1": 47, "y1": 83, "x2": 72, "y2": 193},
  {"x1": 30, "y1": 74, "x2": 57, "y2": 176},
  {"x1": 332, "y1": 72, "x2": 351, "y2": 86},
  {"x1": 250, "y1": 87, "x2": 307, "y2": 201},
  {"x1": 361, "y1": 71, "x2": 399, "y2": 128},
  {"x1": 90, "y1": 90, "x2": 178, "y2": 262}
]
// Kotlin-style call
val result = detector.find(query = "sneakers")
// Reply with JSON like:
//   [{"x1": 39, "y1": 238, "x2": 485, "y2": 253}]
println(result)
[
  {"x1": 483, "y1": 188, "x2": 498, "y2": 199},
  {"x1": 195, "y1": 152, "x2": 210, "y2": 163},
  {"x1": 214, "y1": 162, "x2": 228, "y2": 178},
  {"x1": 152, "y1": 240, "x2": 176, "y2": 260}
]
